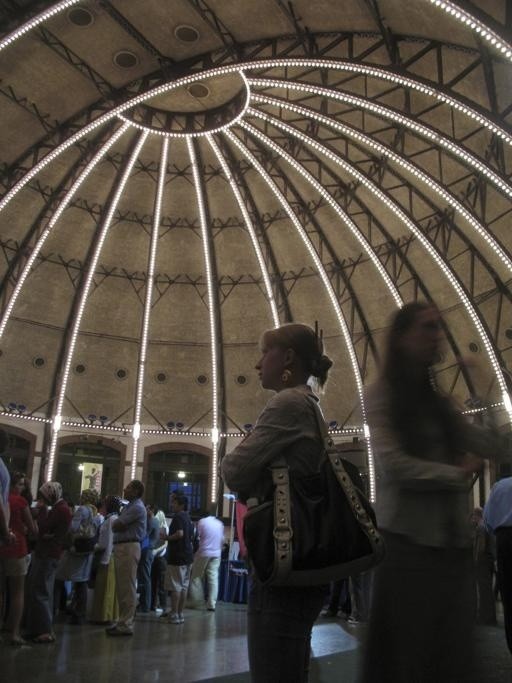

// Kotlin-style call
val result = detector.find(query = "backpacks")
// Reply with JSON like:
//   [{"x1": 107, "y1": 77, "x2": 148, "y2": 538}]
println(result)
[{"x1": 190, "y1": 520, "x2": 200, "y2": 555}]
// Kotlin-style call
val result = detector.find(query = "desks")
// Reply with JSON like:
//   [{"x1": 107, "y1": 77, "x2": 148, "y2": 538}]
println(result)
[{"x1": 218, "y1": 558, "x2": 252, "y2": 605}]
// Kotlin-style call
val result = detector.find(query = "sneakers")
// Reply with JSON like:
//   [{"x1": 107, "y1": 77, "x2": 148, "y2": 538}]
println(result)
[
  {"x1": 159, "y1": 614, "x2": 184, "y2": 624},
  {"x1": 33, "y1": 631, "x2": 55, "y2": 643},
  {"x1": 320, "y1": 610, "x2": 361, "y2": 624},
  {"x1": 105, "y1": 624, "x2": 134, "y2": 636}
]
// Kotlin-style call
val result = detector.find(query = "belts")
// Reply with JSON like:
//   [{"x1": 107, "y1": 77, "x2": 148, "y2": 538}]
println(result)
[{"x1": 112, "y1": 539, "x2": 141, "y2": 545}]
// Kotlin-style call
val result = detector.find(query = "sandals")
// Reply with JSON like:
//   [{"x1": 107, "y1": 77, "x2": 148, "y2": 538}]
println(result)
[{"x1": 5, "y1": 632, "x2": 24, "y2": 645}]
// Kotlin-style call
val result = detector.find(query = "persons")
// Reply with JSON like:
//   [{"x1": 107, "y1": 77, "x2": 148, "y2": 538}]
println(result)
[
  {"x1": 221, "y1": 324, "x2": 331, "y2": 681},
  {"x1": 359, "y1": 300, "x2": 510, "y2": 681}
]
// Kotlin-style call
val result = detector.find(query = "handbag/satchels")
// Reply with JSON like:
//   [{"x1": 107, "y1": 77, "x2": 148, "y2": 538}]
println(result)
[{"x1": 242, "y1": 388, "x2": 386, "y2": 588}]
